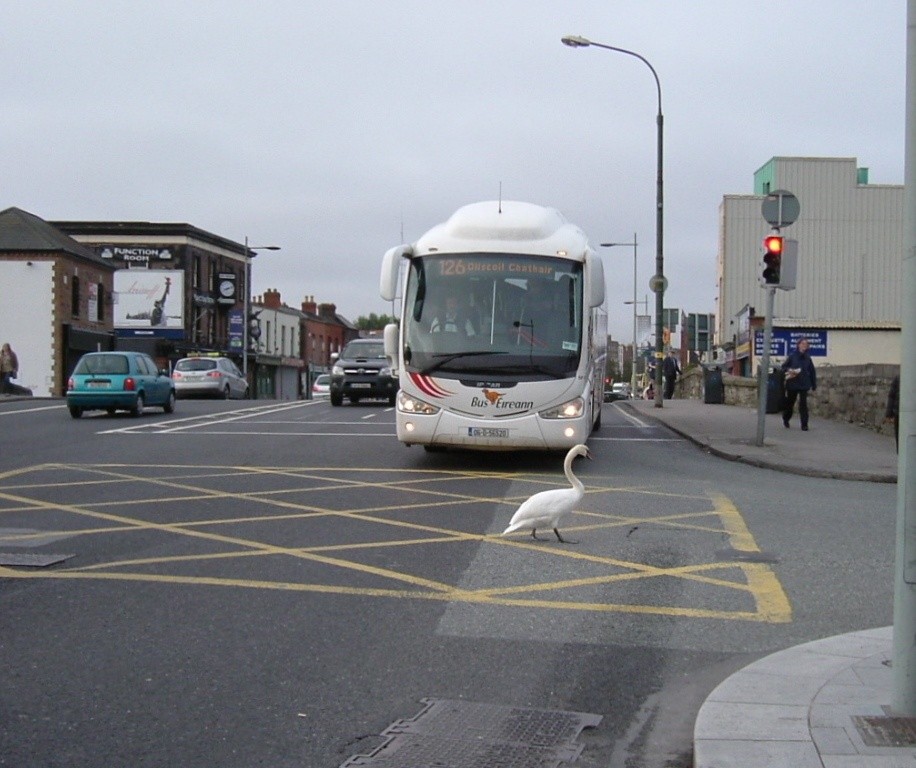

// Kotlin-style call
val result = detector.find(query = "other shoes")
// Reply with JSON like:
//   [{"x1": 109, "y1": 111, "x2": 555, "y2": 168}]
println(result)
[
  {"x1": 784, "y1": 420, "x2": 790, "y2": 428},
  {"x1": 801, "y1": 424, "x2": 808, "y2": 431}
]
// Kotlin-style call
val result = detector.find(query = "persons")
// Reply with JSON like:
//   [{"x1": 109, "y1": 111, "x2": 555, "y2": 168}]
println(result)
[
  {"x1": 430, "y1": 298, "x2": 477, "y2": 337},
  {"x1": 0, "y1": 343, "x2": 18, "y2": 394},
  {"x1": 663, "y1": 349, "x2": 683, "y2": 399},
  {"x1": 781, "y1": 337, "x2": 816, "y2": 432},
  {"x1": 883, "y1": 373, "x2": 900, "y2": 454},
  {"x1": 648, "y1": 358, "x2": 656, "y2": 400}
]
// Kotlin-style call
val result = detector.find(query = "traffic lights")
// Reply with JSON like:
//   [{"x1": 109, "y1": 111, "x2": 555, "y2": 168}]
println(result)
[{"x1": 762, "y1": 233, "x2": 786, "y2": 288}]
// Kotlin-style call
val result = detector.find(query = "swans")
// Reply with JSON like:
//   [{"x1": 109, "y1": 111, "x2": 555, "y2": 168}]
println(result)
[{"x1": 501, "y1": 444, "x2": 593, "y2": 544}]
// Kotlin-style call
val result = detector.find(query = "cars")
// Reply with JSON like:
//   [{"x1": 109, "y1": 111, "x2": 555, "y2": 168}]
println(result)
[
  {"x1": 66, "y1": 350, "x2": 177, "y2": 419},
  {"x1": 604, "y1": 376, "x2": 644, "y2": 403},
  {"x1": 311, "y1": 373, "x2": 331, "y2": 401},
  {"x1": 172, "y1": 356, "x2": 251, "y2": 401}
]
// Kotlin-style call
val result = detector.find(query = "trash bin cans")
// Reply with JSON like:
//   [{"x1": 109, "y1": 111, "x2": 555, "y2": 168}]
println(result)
[
  {"x1": 756, "y1": 363, "x2": 784, "y2": 415},
  {"x1": 703, "y1": 364, "x2": 725, "y2": 404}
]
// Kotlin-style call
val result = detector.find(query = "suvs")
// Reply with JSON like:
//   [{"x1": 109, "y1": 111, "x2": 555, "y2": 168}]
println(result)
[{"x1": 328, "y1": 336, "x2": 400, "y2": 407}]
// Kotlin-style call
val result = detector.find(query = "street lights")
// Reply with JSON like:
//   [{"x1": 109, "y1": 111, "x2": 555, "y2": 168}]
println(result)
[
  {"x1": 623, "y1": 293, "x2": 649, "y2": 389},
  {"x1": 599, "y1": 231, "x2": 638, "y2": 400},
  {"x1": 560, "y1": 33, "x2": 666, "y2": 407},
  {"x1": 512, "y1": 318, "x2": 535, "y2": 363},
  {"x1": 240, "y1": 236, "x2": 283, "y2": 381}
]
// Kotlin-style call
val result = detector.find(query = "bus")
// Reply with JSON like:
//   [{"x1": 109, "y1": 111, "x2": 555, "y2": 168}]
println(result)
[{"x1": 379, "y1": 200, "x2": 609, "y2": 454}]
